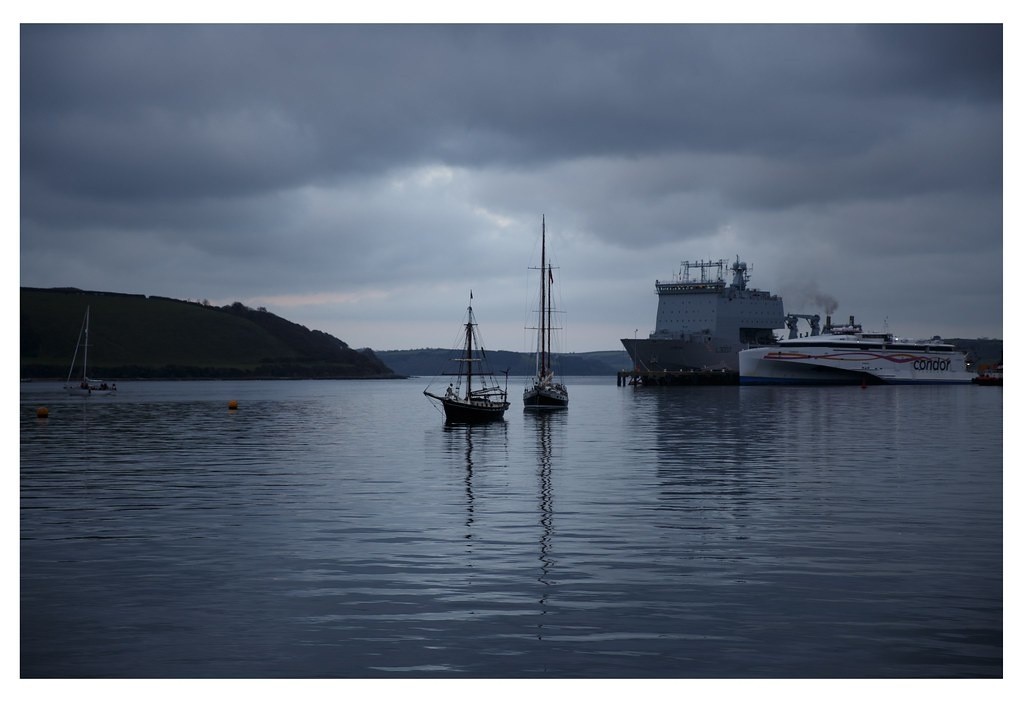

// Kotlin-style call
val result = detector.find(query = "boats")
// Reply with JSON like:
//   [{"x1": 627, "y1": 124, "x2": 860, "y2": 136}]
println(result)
[{"x1": 619, "y1": 253, "x2": 1003, "y2": 387}]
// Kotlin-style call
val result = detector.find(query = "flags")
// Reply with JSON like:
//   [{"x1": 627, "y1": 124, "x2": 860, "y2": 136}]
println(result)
[
  {"x1": 470, "y1": 292, "x2": 473, "y2": 299},
  {"x1": 549, "y1": 267, "x2": 553, "y2": 283}
]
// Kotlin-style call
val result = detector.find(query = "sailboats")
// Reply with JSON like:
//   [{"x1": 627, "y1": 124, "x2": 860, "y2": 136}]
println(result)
[
  {"x1": 63, "y1": 304, "x2": 118, "y2": 397},
  {"x1": 423, "y1": 288, "x2": 511, "y2": 425},
  {"x1": 522, "y1": 212, "x2": 569, "y2": 411}
]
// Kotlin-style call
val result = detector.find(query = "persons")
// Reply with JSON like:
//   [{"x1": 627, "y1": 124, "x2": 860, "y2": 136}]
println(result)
[{"x1": 80, "y1": 381, "x2": 117, "y2": 391}]
